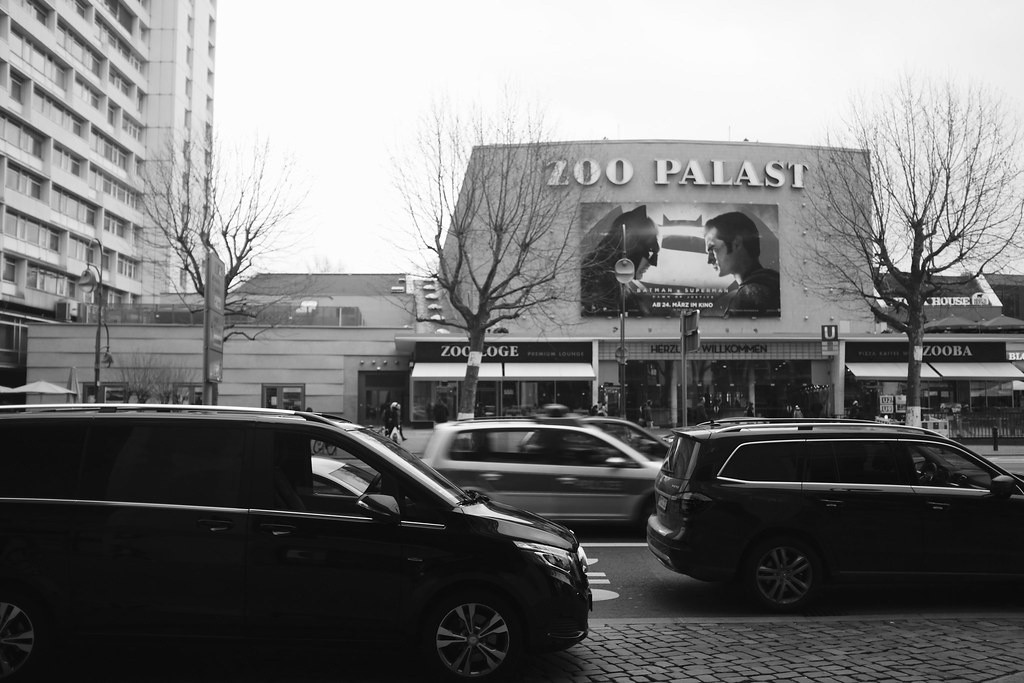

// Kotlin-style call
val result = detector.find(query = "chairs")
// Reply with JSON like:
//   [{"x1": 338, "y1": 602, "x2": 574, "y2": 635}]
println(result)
[
  {"x1": 841, "y1": 446, "x2": 866, "y2": 481},
  {"x1": 872, "y1": 447, "x2": 894, "y2": 480}
]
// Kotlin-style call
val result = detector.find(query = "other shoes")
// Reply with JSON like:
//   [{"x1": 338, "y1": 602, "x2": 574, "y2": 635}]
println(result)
[{"x1": 402, "y1": 438, "x2": 407, "y2": 441}]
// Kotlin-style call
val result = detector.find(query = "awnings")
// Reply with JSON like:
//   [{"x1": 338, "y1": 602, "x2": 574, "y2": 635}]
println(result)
[
  {"x1": 930, "y1": 362, "x2": 1024, "y2": 380},
  {"x1": 505, "y1": 363, "x2": 597, "y2": 380},
  {"x1": 846, "y1": 362, "x2": 942, "y2": 381},
  {"x1": 411, "y1": 363, "x2": 503, "y2": 381}
]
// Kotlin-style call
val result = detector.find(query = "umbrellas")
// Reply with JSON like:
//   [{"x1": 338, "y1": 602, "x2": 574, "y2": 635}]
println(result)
[{"x1": 0, "y1": 380, "x2": 77, "y2": 404}]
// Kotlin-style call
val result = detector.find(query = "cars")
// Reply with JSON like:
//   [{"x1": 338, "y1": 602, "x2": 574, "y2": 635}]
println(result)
[
  {"x1": 519, "y1": 415, "x2": 672, "y2": 460},
  {"x1": 310, "y1": 454, "x2": 375, "y2": 498},
  {"x1": 418, "y1": 402, "x2": 665, "y2": 540}
]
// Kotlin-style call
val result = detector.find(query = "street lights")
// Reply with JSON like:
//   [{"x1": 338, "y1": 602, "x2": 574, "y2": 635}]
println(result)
[
  {"x1": 78, "y1": 238, "x2": 115, "y2": 403},
  {"x1": 615, "y1": 224, "x2": 635, "y2": 421}
]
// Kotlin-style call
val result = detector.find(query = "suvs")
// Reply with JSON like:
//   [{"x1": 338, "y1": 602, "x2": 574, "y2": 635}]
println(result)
[
  {"x1": 646, "y1": 416, "x2": 1023, "y2": 616},
  {"x1": 1, "y1": 403, "x2": 593, "y2": 683}
]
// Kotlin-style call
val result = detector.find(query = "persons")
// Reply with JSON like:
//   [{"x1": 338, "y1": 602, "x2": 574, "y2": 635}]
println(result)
[
  {"x1": 704, "y1": 211, "x2": 780, "y2": 317},
  {"x1": 433, "y1": 398, "x2": 449, "y2": 427},
  {"x1": 694, "y1": 397, "x2": 722, "y2": 424},
  {"x1": 383, "y1": 402, "x2": 407, "y2": 444},
  {"x1": 794, "y1": 405, "x2": 803, "y2": 417},
  {"x1": 640, "y1": 400, "x2": 654, "y2": 427},
  {"x1": 590, "y1": 402, "x2": 608, "y2": 417},
  {"x1": 743, "y1": 403, "x2": 755, "y2": 417},
  {"x1": 945, "y1": 401, "x2": 973, "y2": 436},
  {"x1": 582, "y1": 206, "x2": 660, "y2": 312},
  {"x1": 475, "y1": 402, "x2": 485, "y2": 418},
  {"x1": 850, "y1": 401, "x2": 860, "y2": 418}
]
